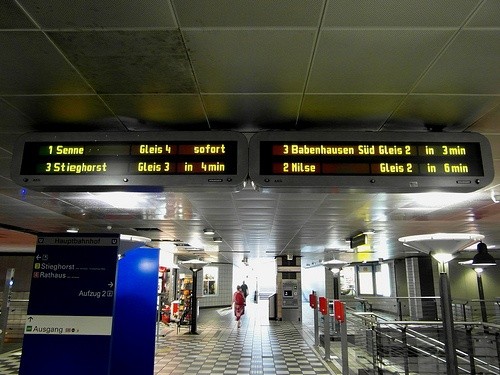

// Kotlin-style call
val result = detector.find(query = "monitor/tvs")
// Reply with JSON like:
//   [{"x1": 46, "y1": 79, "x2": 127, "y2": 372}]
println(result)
[{"x1": 284, "y1": 290, "x2": 292, "y2": 296}]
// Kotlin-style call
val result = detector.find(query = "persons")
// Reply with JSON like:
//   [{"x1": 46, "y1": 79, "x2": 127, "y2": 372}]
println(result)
[
  {"x1": 231, "y1": 285, "x2": 246, "y2": 328},
  {"x1": 241, "y1": 281, "x2": 249, "y2": 298}
]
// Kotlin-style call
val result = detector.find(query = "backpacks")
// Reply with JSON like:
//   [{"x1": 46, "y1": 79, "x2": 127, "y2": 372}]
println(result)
[{"x1": 236, "y1": 293, "x2": 244, "y2": 306}]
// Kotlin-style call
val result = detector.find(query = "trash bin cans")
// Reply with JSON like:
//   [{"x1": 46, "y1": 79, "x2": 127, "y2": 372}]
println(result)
[{"x1": 269, "y1": 292, "x2": 277, "y2": 320}]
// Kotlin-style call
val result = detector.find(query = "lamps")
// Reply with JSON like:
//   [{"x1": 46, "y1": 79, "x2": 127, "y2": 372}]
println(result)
[{"x1": 472, "y1": 241, "x2": 497, "y2": 266}]
